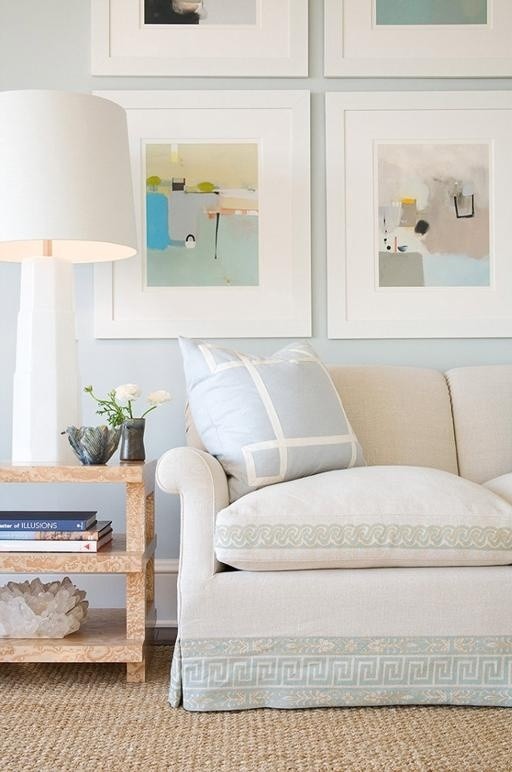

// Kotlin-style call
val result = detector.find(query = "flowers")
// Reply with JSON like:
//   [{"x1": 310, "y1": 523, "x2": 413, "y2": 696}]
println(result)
[{"x1": 83, "y1": 381, "x2": 173, "y2": 423}]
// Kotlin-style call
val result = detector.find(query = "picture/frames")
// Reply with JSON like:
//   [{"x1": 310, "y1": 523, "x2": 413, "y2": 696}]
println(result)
[
  {"x1": 88, "y1": 0, "x2": 314, "y2": 80},
  {"x1": 322, "y1": 90, "x2": 512, "y2": 341},
  {"x1": 319, "y1": 1, "x2": 510, "y2": 81},
  {"x1": 89, "y1": 86, "x2": 315, "y2": 342}
]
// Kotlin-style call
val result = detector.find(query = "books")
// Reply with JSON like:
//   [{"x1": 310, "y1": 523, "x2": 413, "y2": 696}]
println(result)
[
  {"x1": 0, "y1": 520, "x2": 114, "y2": 541},
  {"x1": 0, "y1": 532, "x2": 114, "y2": 553},
  {"x1": 0, "y1": 511, "x2": 98, "y2": 531}
]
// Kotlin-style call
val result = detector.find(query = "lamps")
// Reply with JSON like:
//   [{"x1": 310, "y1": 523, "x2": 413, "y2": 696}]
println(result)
[{"x1": 2, "y1": 85, "x2": 140, "y2": 467}]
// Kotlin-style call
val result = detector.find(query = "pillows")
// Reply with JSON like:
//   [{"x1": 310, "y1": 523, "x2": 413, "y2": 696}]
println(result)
[{"x1": 175, "y1": 334, "x2": 372, "y2": 507}]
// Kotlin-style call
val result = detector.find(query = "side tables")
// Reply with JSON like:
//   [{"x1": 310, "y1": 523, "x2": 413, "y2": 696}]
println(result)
[{"x1": 1, "y1": 452, "x2": 162, "y2": 690}]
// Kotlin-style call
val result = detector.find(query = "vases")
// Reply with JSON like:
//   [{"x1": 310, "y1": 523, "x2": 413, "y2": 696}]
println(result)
[{"x1": 116, "y1": 416, "x2": 148, "y2": 463}]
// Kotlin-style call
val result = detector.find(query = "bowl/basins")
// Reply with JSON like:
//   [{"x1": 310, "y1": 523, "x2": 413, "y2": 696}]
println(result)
[{"x1": 65, "y1": 426, "x2": 122, "y2": 463}]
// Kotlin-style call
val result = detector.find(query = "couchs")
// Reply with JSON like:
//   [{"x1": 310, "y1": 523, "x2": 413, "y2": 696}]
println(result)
[{"x1": 153, "y1": 360, "x2": 512, "y2": 716}]
